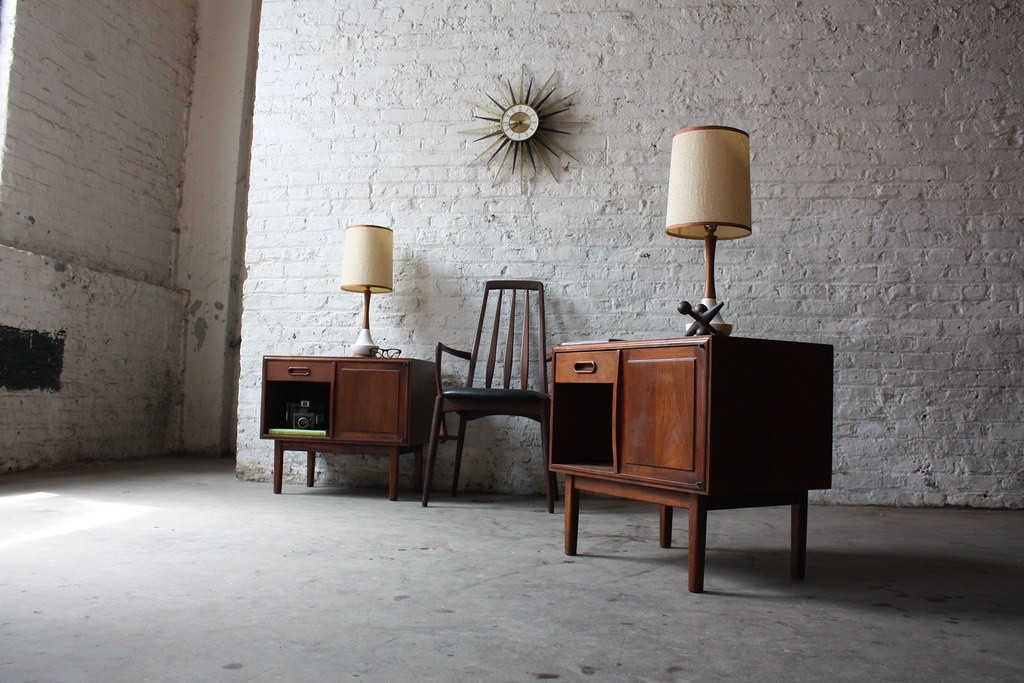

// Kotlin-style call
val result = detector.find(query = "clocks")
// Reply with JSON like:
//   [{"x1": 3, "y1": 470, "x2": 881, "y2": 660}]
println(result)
[{"x1": 457, "y1": 62, "x2": 590, "y2": 195}]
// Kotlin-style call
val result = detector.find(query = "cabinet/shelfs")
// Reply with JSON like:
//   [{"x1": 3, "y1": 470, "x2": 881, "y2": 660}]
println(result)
[
  {"x1": 548, "y1": 335, "x2": 834, "y2": 592},
  {"x1": 259, "y1": 355, "x2": 438, "y2": 501}
]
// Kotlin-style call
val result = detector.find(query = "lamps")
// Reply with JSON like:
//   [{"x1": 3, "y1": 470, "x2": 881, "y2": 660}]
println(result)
[
  {"x1": 665, "y1": 125, "x2": 752, "y2": 336},
  {"x1": 341, "y1": 224, "x2": 393, "y2": 357}
]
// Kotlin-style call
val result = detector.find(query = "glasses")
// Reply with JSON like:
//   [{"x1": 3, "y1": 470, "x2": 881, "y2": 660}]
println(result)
[{"x1": 369, "y1": 348, "x2": 401, "y2": 359}]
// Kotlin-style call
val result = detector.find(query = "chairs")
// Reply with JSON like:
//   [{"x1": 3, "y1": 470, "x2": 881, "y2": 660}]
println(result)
[{"x1": 422, "y1": 280, "x2": 560, "y2": 513}]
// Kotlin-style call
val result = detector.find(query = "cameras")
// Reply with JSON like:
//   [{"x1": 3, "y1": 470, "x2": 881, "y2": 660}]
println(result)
[{"x1": 293, "y1": 401, "x2": 325, "y2": 430}]
